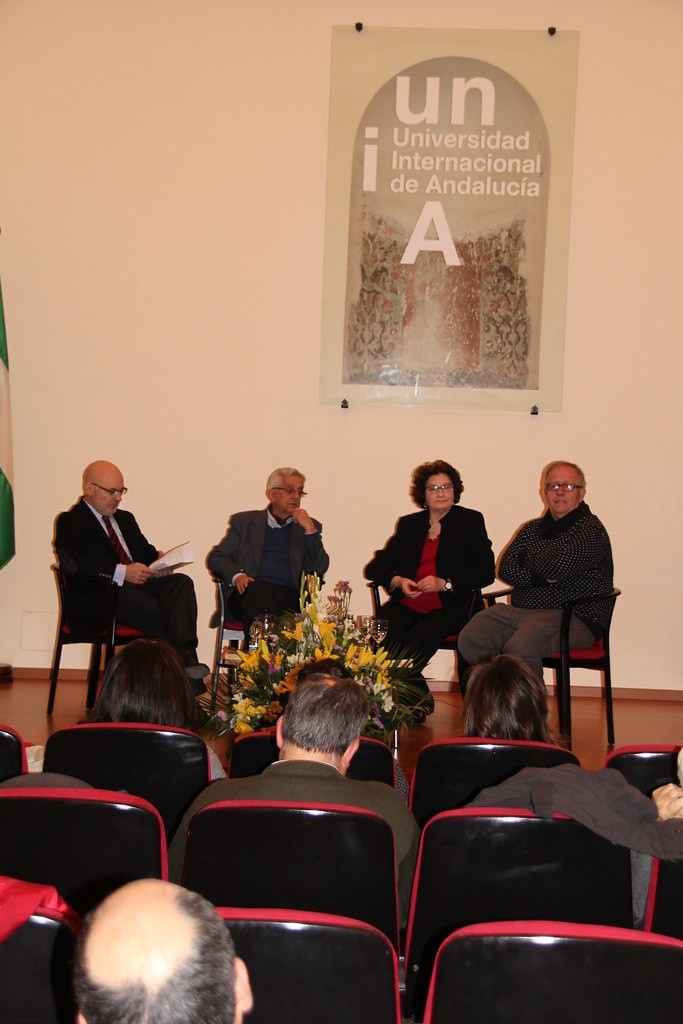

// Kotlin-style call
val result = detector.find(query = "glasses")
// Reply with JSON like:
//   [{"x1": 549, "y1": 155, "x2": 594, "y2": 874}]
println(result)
[
  {"x1": 272, "y1": 486, "x2": 308, "y2": 498},
  {"x1": 426, "y1": 483, "x2": 453, "y2": 493},
  {"x1": 546, "y1": 482, "x2": 583, "y2": 492},
  {"x1": 90, "y1": 483, "x2": 128, "y2": 496}
]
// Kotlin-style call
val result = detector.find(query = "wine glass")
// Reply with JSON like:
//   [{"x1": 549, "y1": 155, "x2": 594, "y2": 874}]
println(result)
[
  {"x1": 249, "y1": 613, "x2": 275, "y2": 654},
  {"x1": 371, "y1": 620, "x2": 388, "y2": 661},
  {"x1": 356, "y1": 615, "x2": 373, "y2": 654}
]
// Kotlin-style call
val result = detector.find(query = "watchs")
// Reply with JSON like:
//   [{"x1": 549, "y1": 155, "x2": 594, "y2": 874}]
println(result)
[{"x1": 444, "y1": 579, "x2": 452, "y2": 592}]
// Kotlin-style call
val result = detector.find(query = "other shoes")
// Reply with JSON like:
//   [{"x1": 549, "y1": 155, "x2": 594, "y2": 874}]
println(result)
[
  {"x1": 405, "y1": 701, "x2": 435, "y2": 726},
  {"x1": 186, "y1": 703, "x2": 211, "y2": 732},
  {"x1": 180, "y1": 650, "x2": 210, "y2": 680}
]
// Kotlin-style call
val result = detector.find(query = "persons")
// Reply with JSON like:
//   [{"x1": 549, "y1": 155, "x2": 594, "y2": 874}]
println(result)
[
  {"x1": 364, "y1": 460, "x2": 495, "y2": 723},
  {"x1": 167, "y1": 674, "x2": 423, "y2": 933},
  {"x1": 457, "y1": 461, "x2": 613, "y2": 681},
  {"x1": 55, "y1": 461, "x2": 209, "y2": 700},
  {"x1": 206, "y1": 468, "x2": 328, "y2": 631},
  {"x1": 631, "y1": 747, "x2": 683, "y2": 927},
  {"x1": 77, "y1": 879, "x2": 252, "y2": 1024},
  {"x1": 299, "y1": 659, "x2": 410, "y2": 804},
  {"x1": 461, "y1": 654, "x2": 567, "y2": 747},
  {"x1": 85, "y1": 638, "x2": 228, "y2": 780}
]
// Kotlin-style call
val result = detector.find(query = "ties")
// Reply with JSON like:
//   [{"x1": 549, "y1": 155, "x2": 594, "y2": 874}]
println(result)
[{"x1": 102, "y1": 515, "x2": 132, "y2": 566}]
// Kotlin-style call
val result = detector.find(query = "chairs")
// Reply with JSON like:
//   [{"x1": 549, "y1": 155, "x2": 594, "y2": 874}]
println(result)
[
  {"x1": 223, "y1": 724, "x2": 398, "y2": 807},
  {"x1": 0, "y1": 721, "x2": 31, "y2": 783},
  {"x1": 419, "y1": 916, "x2": 683, "y2": 1024},
  {"x1": 641, "y1": 817, "x2": 683, "y2": 941},
  {"x1": 45, "y1": 562, "x2": 147, "y2": 718},
  {"x1": 210, "y1": 903, "x2": 406, "y2": 1024},
  {"x1": 365, "y1": 581, "x2": 481, "y2": 706},
  {"x1": 405, "y1": 730, "x2": 594, "y2": 833},
  {"x1": 0, "y1": 886, "x2": 80, "y2": 1024},
  {"x1": 181, "y1": 796, "x2": 405, "y2": 996},
  {"x1": 0, "y1": 785, "x2": 174, "y2": 918},
  {"x1": 399, "y1": 805, "x2": 642, "y2": 1017},
  {"x1": 40, "y1": 720, "x2": 214, "y2": 841},
  {"x1": 597, "y1": 742, "x2": 683, "y2": 800},
  {"x1": 206, "y1": 558, "x2": 326, "y2": 718},
  {"x1": 482, "y1": 578, "x2": 630, "y2": 751}
]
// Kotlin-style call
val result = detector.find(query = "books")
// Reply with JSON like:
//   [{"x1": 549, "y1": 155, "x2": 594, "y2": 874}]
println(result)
[{"x1": 150, "y1": 541, "x2": 194, "y2": 570}]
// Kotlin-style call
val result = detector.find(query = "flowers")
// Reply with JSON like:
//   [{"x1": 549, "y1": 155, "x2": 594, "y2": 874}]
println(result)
[{"x1": 196, "y1": 569, "x2": 451, "y2": 750}]
